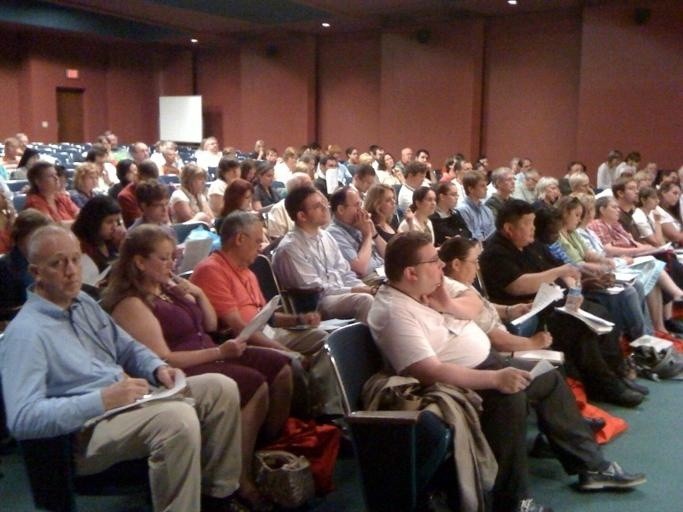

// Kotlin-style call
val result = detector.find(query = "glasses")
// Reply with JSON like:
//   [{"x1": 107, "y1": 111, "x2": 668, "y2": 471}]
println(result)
[
  {"x1": 412, "y1": 256, "x2": 439, "y2": 267},
  {"x1": 459, "y1": 258, "x2": 480, "y2": 267}
]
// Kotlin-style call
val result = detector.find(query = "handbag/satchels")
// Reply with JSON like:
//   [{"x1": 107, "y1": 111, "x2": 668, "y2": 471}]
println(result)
[{"x1": 252, "y1": 448, "x2": 315, "y2": 511}]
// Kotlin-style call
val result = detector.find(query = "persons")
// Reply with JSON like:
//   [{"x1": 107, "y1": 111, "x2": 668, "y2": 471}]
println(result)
[
  {"x1": 513, "y1": 158, "x2": 594, "y2": 199},
  {"x1": 532, "y1": 207, "x2": 645, "y2": 339},
  {"x1": 429, "y1": 238, "x2": 606, "y2": 433},
  {"x1": 188, "y1": 211, "x2": 328, "y2": 444},
  {"x1": 587, "y1": 197, "x2": 652, "y2": 256},
  {"x1": 481, "y1": 200, "x2": 649, "y2": 408},
  {"x1": 102, "y1": 225, "x2": 293, "y2": 505},
  {"x1": 550, "y1": 197, "x2": 654, "y2": 300},
  {"x1": 657, "y1": 183, "x2": 683, "y2": 249},
  {"x1": 1, "y1": 225, "x2": 262, "y2": 512},
  {"x1": 430, "y1": 181, "x2": 472, "y2": 247},
  {"x1": 457, "y1": 171, "x2": 496, "y2": 242},
  {"x1": 596, "y1": 148, "x2": 683, "y2": 177},
  {"x1": 632, "y1": 187, "x2": 683, "y2": 262},
  {"x1": 271, "y1": 186, "x2": 377, "y2": 323},
  {"x1": 0, "y1": 131, "x2": 273, "y2": 226},
  {"x1": 610, "y1": 177, "x2": 683, "y2": 334},
  {"x1": 274, "y1": 144, "x2": 491, "y2": 182},
  {"x1": 364, "y1": 183, "x2": 401, "y2": 241},
  {"x1": 397, "y1": 187, "x2": 437, "y2": 247},
  {"x1": 483, "y1": 166, "x2": 517, "y2": 218},
  {"x1": 367, "y1": 231, "x2": 648, "y2": 512},
  {"x1": 327, "y1": 186, "x2": 388, "y2": 286},
  {"x1": 568, "y1": 193, "x2": 683, "y2": 339}
]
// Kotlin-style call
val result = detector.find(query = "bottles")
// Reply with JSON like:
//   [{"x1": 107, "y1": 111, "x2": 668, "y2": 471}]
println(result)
[{"x1": 565, "y1": 277, "x2": 583, "y2": 315}]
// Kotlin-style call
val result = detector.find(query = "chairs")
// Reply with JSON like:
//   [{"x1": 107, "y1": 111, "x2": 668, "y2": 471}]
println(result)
[
  {"x1": 249, "y1": 254, "x2": 288, "y2": 314},
  {"x1": 171, "y1": 221, "x2": 210, "y2": 246},
  {"x1": 12, "y1": 193, "x2": 26, "y2": 214},
  {"x1": 323, "y1": 321, "x2": 451, "y2": 512}
]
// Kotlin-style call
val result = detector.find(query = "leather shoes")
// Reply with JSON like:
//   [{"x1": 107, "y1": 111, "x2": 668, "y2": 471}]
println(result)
[
  {"x1": 591, "y1": 377, "x2": 644, "y2": 409},
  {"x1": 577, "y1": 461, "x2": 649, "y2": 491},
  {"x1": 583, "y1": 414, "x2": 606, "y2": 434},
  {"x1": 618, "y1": 372, "x2": 651, "y2": 394},
  {"x1": 514, "y1": 497, "x2": 556, "y2": 512}
]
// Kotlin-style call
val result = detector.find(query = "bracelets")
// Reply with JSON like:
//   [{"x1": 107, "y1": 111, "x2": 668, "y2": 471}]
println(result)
[{"x1": 216, "y1": 347, "x2": 221, "y2": 363}]
